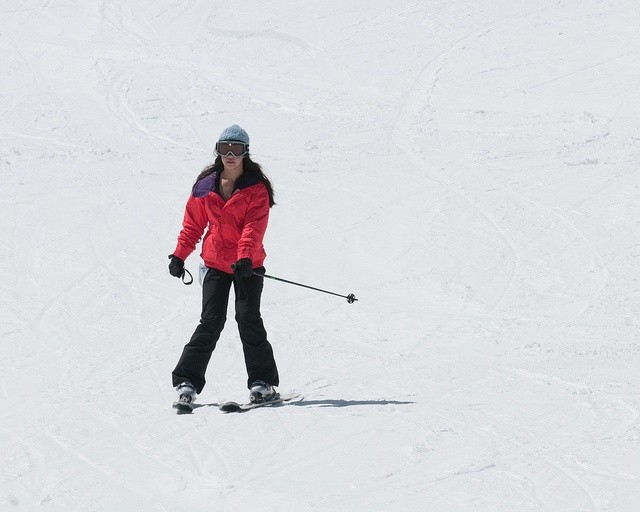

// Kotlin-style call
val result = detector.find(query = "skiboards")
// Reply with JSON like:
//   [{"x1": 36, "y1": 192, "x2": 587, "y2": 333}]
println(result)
[{"x1": 173, "y1": 389, "x2": 300, "y2": 413}]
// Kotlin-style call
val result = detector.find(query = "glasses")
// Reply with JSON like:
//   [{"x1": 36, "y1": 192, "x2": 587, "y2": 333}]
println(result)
[{"x1": 216, "y1": 141, "x2": 249, "y2": 158}]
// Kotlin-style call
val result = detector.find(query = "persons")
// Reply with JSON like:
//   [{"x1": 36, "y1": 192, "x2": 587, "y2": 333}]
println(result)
[{"x1": 169, "y1": 125, "x2": 279, "y2": 401}]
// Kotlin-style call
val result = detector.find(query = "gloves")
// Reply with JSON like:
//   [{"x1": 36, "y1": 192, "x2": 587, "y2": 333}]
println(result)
[
  {"x1": 169, "y1": 257, "x2": 185, "y2": 278},
  {"x1": 236, "y1": 259, "x2": 252, "y2": 277}
]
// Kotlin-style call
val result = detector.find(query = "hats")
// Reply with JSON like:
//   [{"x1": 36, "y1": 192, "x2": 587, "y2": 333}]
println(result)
[{"x1": 219, "y1": 124, "x2": 249, "y2": 142}]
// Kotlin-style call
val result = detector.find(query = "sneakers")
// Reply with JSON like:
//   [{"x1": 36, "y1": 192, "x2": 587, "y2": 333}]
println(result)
[
  {"x1": 177, "y1": 381, "x2": 196, "y2": 400},
  {"x1": 251, "y1": 381, "x2": 272, "y2": 402}
]
